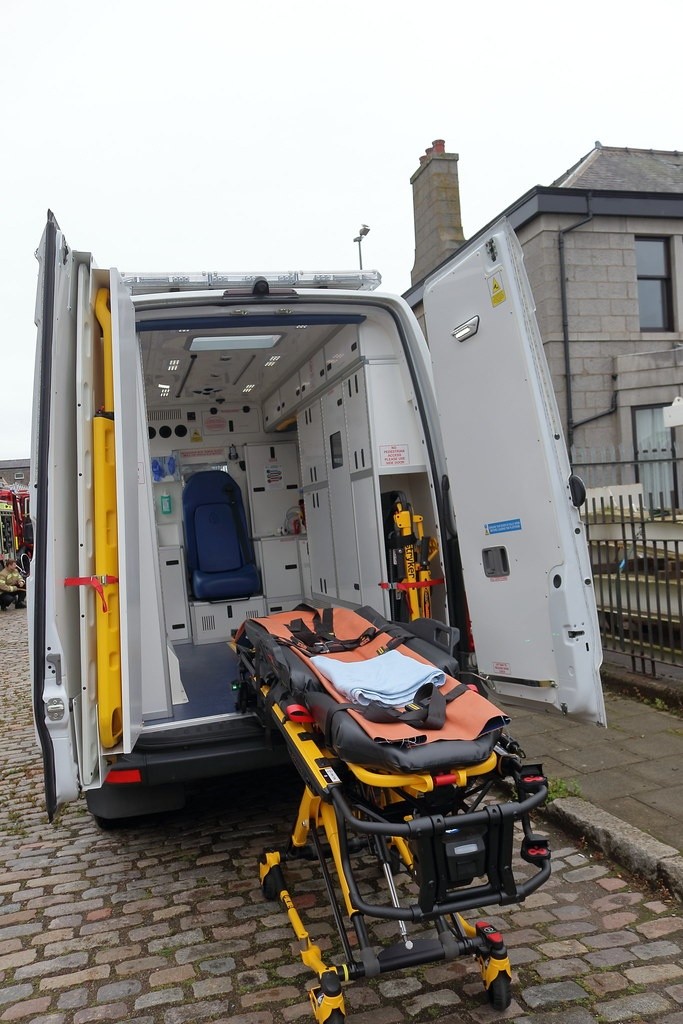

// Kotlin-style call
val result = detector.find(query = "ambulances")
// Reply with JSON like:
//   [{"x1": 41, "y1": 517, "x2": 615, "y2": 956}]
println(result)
[{"x1": 25, "y1": 205, "x2": 610, "y2": 832}]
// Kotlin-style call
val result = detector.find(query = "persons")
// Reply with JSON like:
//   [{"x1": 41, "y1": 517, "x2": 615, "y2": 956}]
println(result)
[
  {"x1": 0, "y1": 558, "x2": 26, "y2": 611},
  {"x1": 16, "y1": 546, "x2": 30, "y2": 576}
]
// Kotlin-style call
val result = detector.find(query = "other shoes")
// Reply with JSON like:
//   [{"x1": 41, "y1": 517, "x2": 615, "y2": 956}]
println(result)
[
  {"x1": 15, "y1": 602, "x2": 27, "y2": 608},
  {"x1": 1, "y1": 605, "x2": 7, "y2": 610}
]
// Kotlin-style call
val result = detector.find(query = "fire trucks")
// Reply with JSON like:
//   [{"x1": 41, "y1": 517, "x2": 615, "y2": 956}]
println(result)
[{"x1": 0, "y1": 476, "x2": 34, "y2": 581}]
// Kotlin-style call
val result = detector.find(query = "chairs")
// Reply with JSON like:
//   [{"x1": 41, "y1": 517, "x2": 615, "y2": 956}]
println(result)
[{"x1": 181, "y1": 470, "x2": 261, "y2": 601}]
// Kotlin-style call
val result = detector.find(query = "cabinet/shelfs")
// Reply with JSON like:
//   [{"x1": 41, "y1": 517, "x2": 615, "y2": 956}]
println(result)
[
  {"x1": 294, "y1": 359, "x2": 450, "y2": 625},
  {"x1": 261, "y1": 321, "x2": 397, "y2": 434},
  {"x1": 257, "y1": 534, "x2": 306, "y2": 615},
  {"x1": 242, "y1": 440, "x2": 303, "y2": 540},
  {"x1": 158, "y1": 545, "x2": 192, "y2": 645}
]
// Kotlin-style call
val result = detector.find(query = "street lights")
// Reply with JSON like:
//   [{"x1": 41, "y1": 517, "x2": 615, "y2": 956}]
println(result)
[{"x1": 352, "y1": 224, "x2": 370, "y2": 271}]
